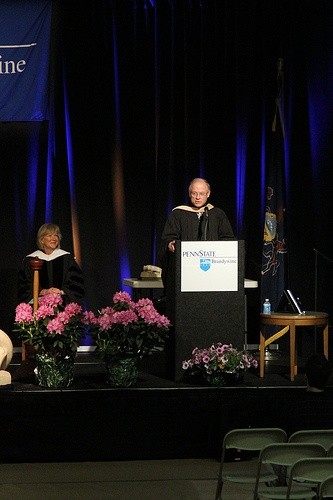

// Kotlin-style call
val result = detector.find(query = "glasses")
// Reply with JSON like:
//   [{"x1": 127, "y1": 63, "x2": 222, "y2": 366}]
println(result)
[{"x1": 190, "y1": 191, "x2": 208, "y2": 197}]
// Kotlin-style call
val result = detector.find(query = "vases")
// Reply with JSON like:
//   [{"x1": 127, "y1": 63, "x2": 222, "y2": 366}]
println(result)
[
  {"x1": 36, "y1": 353, "x2": 76, "y2": 389},
  {"x1": 205, "y1": 373, "x2": 243, "y2": 386},
  {"x1": 100, "y1": 350, "x2": 141, "y2": 388}
]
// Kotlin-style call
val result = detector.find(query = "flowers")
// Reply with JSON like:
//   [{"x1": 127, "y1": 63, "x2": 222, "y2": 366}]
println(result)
[
  {"x1": 182, "y1": 343, "x2": 258, "y2": 373},
  {"x1": 14, "y1": 288, "x2": 87, "y2": 352},
  {"x1": 80, "y1": 291, "x2": 174, "y2": 360}
]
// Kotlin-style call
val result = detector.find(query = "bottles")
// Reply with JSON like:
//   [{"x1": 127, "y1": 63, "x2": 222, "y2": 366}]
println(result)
[{"x1": 263, "y1": 299, "x2": 271, "y2": 316}]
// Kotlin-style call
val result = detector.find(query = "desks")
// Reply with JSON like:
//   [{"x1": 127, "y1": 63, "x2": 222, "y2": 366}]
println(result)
[{"x1": 261, "y1": 311, "x2": 328, "y2": 381}]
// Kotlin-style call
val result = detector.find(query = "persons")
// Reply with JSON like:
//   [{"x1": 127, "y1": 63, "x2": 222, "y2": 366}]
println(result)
[
  {"x1": 20, "y1": 223, "x2": 84, "y2": 313},
  {"x1": 159, "y1": 177, "x2": 234, "y2": 259}
]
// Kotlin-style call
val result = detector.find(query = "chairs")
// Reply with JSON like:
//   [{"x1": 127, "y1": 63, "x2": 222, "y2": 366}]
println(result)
[{"x1": 215, "y1": 428, "x2": 333, "y2": 500}]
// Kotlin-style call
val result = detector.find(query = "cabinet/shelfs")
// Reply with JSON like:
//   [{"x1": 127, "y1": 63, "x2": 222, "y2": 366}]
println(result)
[{"x1": 124, "y1": 278, "x2": 258, "y2": 359}]
